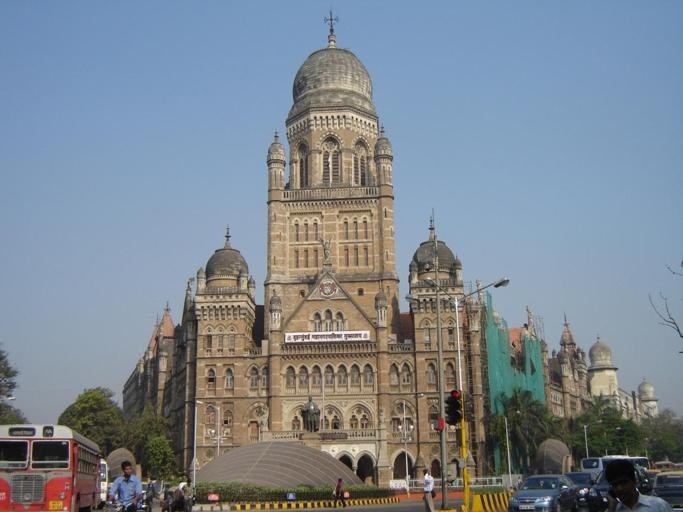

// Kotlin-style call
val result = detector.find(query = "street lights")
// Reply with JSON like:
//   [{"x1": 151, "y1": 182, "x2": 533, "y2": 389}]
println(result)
[
  {"x1": 604, "y1": 427, "x2": 621, "y2": 456},
  {"x1": 584, "y1": 420, "x2": 602, "y2": 458},
  {"x1": 195, "y1": 401, "x2": 227, "y2": 457},
  {"x1": 505, "y1": 410, "x2": 520, "y2": 487},
  {"x1": 398, "y1": 393, "x2": 425, "y2": 493},
  {"x1": 423, "y1": 277, "x2": 509, "y2": 391}
]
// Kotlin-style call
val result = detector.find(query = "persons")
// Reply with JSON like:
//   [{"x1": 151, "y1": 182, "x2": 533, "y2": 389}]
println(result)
[
  {"x1": 106, "y1": 460, "x2": 143, "y2": 511},
  {"x1": 323, "y1": 239, "x2": 332, "y2": 262},
  {"x1": 147, "y1": 481, "x2": 153, "y2": 488},
  {"x1": 300, "y1": 395, "x2": 320, "y2": 432},
  {"x1": 604, "y1": 459, "x2": 674, "y2": 512},
  {"x1": 160, "y1": 478, "x2": 194, "y2": 512},
  {"x1": 422, "y1": 469, "x2": 436, "y2": 512},
  {"x1": 333, "y1": 478, "x2": 347, "y2": 508}
]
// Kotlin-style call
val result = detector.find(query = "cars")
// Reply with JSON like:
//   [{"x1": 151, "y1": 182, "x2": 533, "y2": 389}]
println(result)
[{"x1": 508, "y1": 467, "x2": 683, "y2": 512}]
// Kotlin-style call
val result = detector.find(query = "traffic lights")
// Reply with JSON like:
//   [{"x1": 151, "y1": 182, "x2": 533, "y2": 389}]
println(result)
[{"x1": 445, "y1": 390, "x2": 475, "y2": 425}]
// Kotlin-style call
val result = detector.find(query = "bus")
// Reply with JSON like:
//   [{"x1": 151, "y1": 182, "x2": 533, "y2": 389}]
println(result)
[
  {"x1": 582, "y1": 456, "x2": 650, "y2": 482},
  {"x1": 0, "y1": 424, "x2": 108, "y2": 511}
]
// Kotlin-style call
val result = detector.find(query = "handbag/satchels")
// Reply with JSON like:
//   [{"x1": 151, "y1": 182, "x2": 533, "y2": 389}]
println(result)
[
  {"x1": 431, "y1": 490, "x2": 435, "y2": 498},
  {"x1": 332, "y1": 490, "x2": 336, "y2": 496}
]
